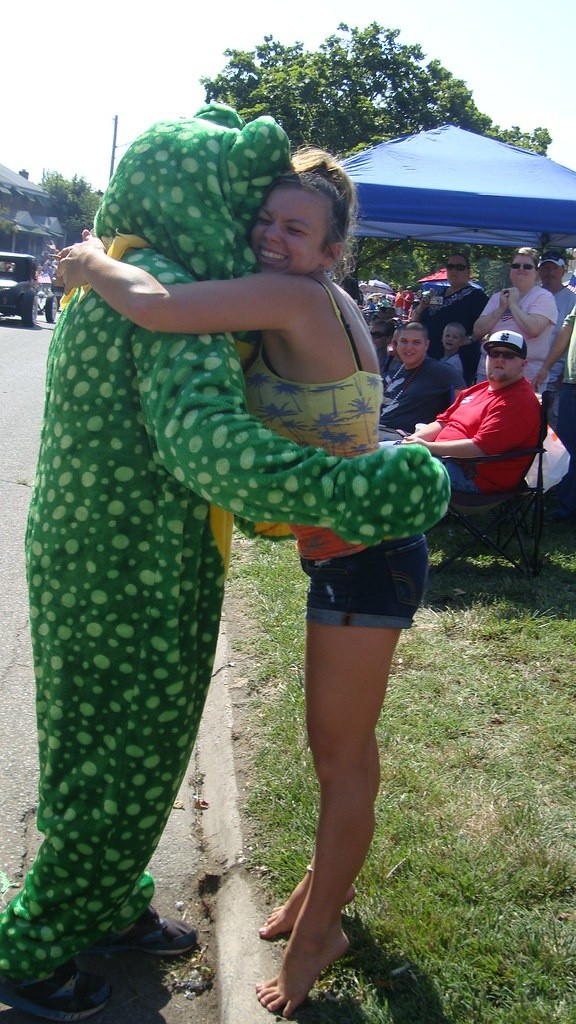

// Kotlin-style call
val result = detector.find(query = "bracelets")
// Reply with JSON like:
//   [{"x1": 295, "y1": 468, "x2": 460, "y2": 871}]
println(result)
[
  {"x1": 413, "y1": 309, "x2": 421, "y2": 316},
  {"x1": 492, "y1": 310, "x2": 498, "y2": 319}
]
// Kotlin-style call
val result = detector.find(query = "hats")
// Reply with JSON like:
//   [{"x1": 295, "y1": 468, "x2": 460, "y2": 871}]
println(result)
[
  {"x1": 482, "y1": 329, "x2": 528, "y2": 360},
  {"x1": 538, "y1": 251, "x2": 566, "y2": 268}
]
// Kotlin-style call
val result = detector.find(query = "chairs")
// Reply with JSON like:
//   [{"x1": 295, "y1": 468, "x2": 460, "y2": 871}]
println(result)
[{"x1": 428, "y1": 389, "x2": 554, "y2": 579}]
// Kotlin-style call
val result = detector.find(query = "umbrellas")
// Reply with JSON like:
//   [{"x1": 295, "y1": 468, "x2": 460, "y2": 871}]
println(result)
[
  {"x1": 362, "y1": 279, "x2": 392, "y2": 294},
  {"x1": 417, "y1": 268, "x2": 482, "y2": 292}
]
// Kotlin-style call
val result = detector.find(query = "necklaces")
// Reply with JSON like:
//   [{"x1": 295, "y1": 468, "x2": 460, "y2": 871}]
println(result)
[{"x1": 381, "y1": 353, "x2": 427, "y2": 417}]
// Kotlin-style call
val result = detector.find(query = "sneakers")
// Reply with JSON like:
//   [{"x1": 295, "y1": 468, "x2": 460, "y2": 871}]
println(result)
[
  {"x1": 79, "y1": 903, "x2": 197, "y2": 954},
  {"x1": 0, "y1": 961, "x2": 114, "y2": 1022}
]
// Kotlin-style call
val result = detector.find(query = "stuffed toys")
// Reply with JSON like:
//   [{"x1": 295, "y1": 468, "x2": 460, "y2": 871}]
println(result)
[{"x1": 0, "y1": 104, "x2": 451, "y2": 1022}]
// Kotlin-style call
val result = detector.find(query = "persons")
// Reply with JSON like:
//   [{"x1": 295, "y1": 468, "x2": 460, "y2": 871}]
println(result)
[
  {"x1": 379, "y1": 321, "x2": 466, "y2": 439},
  {"x1": 5, "y1": 263, "x2": 15, "y2": 272},
  {"x1": 532, "y1": 303, "x2": 576, "y2": 525},
  {"x1": 403, "y1": 330, "x2": 542, "y2": 493},
  {"x1": 331, "y1": 258, "x2": 363, "y2": 306},
  {"x1": 367, "y1": 307, "x2": 396, "y2": 369},
  {"x1": 473, "y1": 246, "x2": 558, "y2": 395},
  {"x1": 537, "y1": 251, "x2": 576, "y2": 392},
  {"x1": 360, "y1": 284, "x2": 423, "y2": 317},
  {"x1": 438, "y1": 322, "x2": 466, "y2": 387},
  {"x1": 56, "y1": 151, "x2": 429, "y2": 1017},
  {"x1": 411, "y1": 254, "x2": 489, "y2": 386},
  {"x1": 36, "y1": 260, "x2": 58, "y2": 315}
]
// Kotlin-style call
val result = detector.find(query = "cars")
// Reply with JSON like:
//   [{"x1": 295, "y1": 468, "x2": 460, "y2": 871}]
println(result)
[{"x1": 0, "y1": 252, "x2": 59, "y2": 327}]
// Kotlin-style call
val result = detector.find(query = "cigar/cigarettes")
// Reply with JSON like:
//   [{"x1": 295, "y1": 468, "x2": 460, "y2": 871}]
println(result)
[{"x1": 48, "y1": 254, "x2": 61, "y2": 260}]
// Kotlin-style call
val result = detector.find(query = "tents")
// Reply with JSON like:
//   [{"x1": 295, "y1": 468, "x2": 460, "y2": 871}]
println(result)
[{"x1": 336, "y1": 125, "x2": 576, "y2": 270}]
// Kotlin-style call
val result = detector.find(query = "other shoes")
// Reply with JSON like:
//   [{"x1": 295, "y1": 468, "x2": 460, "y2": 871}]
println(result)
[{"x1": 543, "y1": 510, "x2": 576, "y2": 523}]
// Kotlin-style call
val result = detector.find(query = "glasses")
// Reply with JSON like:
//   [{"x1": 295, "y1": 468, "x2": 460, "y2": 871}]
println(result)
[
  {"x1": 511, "y1": 263, "x2": 538, "y2": 270},
  {"x1": 369, "y1": 331, "x2": 391, "y2": 339},
  {"x1": 487, "y1": 350, "x2": 526, "y2": 360},
  {"x1": 445, "y1": 264, "x2": 468, "y2": 271}
]
